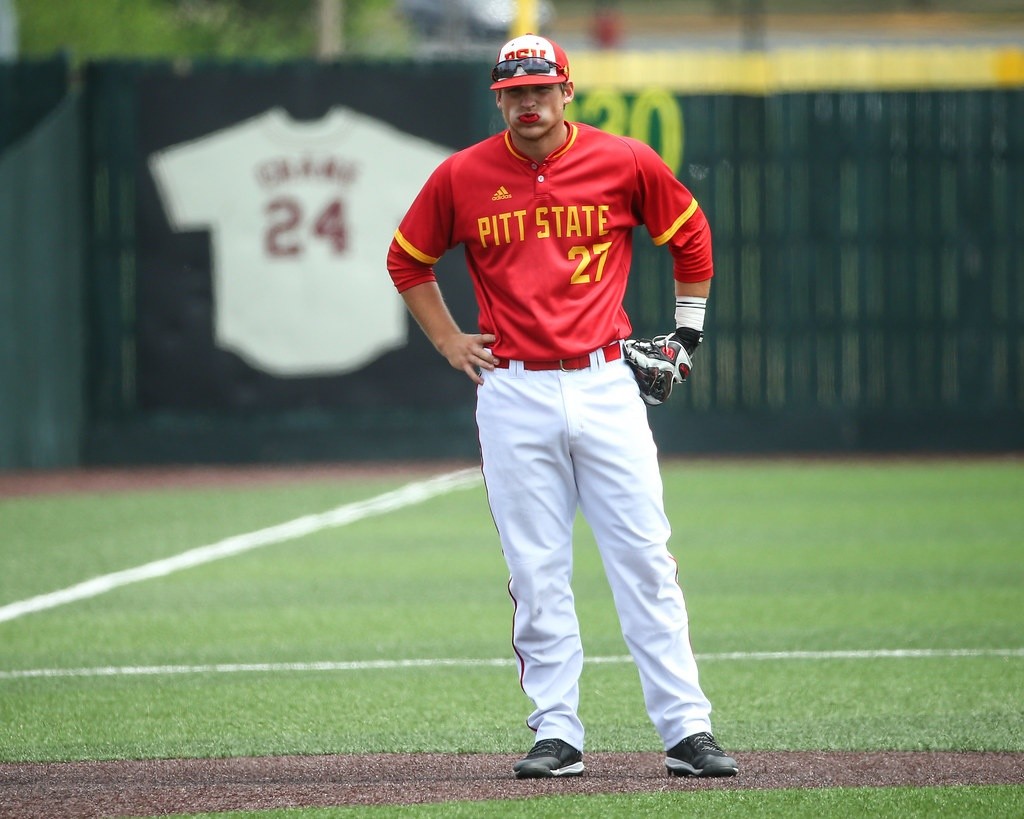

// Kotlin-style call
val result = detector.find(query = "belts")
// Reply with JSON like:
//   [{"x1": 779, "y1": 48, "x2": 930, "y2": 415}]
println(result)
[{"x1": 493, "y1": 342, "x2": 621, "y2": 372}]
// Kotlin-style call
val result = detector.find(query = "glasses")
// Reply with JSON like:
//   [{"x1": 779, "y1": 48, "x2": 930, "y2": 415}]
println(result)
[{"x1": 491, "y1": 56, "x2": 568, "y2": 82}]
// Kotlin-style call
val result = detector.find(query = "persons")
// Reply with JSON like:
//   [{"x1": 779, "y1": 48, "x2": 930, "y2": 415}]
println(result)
[{"x1": 387, "y1": 32, "x2": 739, "y2": 780}]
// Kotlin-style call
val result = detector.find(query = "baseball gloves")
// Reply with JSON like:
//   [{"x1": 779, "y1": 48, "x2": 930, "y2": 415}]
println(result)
[{"x1": 622, "y1": 332, "x2": 695, "y2": 407}]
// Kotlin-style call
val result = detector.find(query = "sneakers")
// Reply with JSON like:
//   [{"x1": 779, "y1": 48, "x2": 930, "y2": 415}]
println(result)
[
  {"x1": 665, "y1": 732, "x2": 738, "y2": 776},
  {"x1": 512, "y1": 739, "x2": 585, "y2": 778}
]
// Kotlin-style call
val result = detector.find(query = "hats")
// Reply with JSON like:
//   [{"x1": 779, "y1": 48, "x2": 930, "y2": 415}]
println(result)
[{"x1": 490, "y1": 32, "x2": 570, "y2": 90}]
